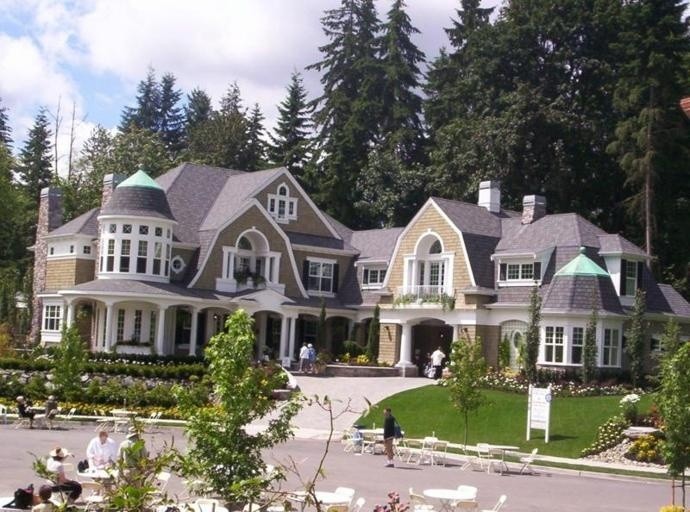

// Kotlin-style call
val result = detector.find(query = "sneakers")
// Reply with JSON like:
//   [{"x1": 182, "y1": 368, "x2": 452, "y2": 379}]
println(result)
[{"x1": 385, "y1": 464, "x2": 394, "y2": 467}]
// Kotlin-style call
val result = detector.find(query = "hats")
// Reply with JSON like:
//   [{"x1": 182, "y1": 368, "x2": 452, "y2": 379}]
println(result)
[
  {"x1": 50, "y1": 447, "x2": 76, "y2": 459},
  {"x1": 126, "y1": 426, "x2": 138, "y2": 439}
]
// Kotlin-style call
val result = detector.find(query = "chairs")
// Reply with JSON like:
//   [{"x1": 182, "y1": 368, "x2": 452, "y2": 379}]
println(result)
[
  {"x1": 344, "y1": 425, "x2": 540, "y2": 477},
  {"x1": 0, "y1": 404, "x2": 163, "y2": 435},
  {"x1": 406, "y1": 485, "x2": 507, "y2": 512}
]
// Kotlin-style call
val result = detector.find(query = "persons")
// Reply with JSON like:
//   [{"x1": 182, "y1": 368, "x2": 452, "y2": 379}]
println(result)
[
  {"x1": 307, "y1": 343, "x2": 320, "y2": 375},
  {"x1": 118, "y1": 426, "x2": 151, "y2": 489},
  {"x1": 45, "y1": 446, "x2": 82, "y2": 506},
  {"x1": 299, "y1": 342, "x2": 310, "y2": 374},
  {"x1": 31, "y1": 483, "x2": 60, "y2": 511},
  {"x1": 430, "y1": 345, "x2": 447, "y2": 379},
  {"x1": 17, "y1": 395, "x2": 37, "y2": 429},
  {"x1": 43, "y1": 394, "x2": 58, "y2": 430},
  {"x1": 383, "y1": 407, "x2": 396, "y2": 467},
  {"x1": 86, "y1": 431, "x2": 117, "y2": 495}
]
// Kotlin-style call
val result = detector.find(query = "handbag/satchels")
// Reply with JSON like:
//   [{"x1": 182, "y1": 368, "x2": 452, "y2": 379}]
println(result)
[
  {"x1": 14, "y1": 484, "x2": 33, "y2": 508},
  {"x1": 394, "y1": 423, "x2": 401, "y2": 438},
  {"x1": 78, "y1": 460, "x2": 89, "y2": 473}
]
